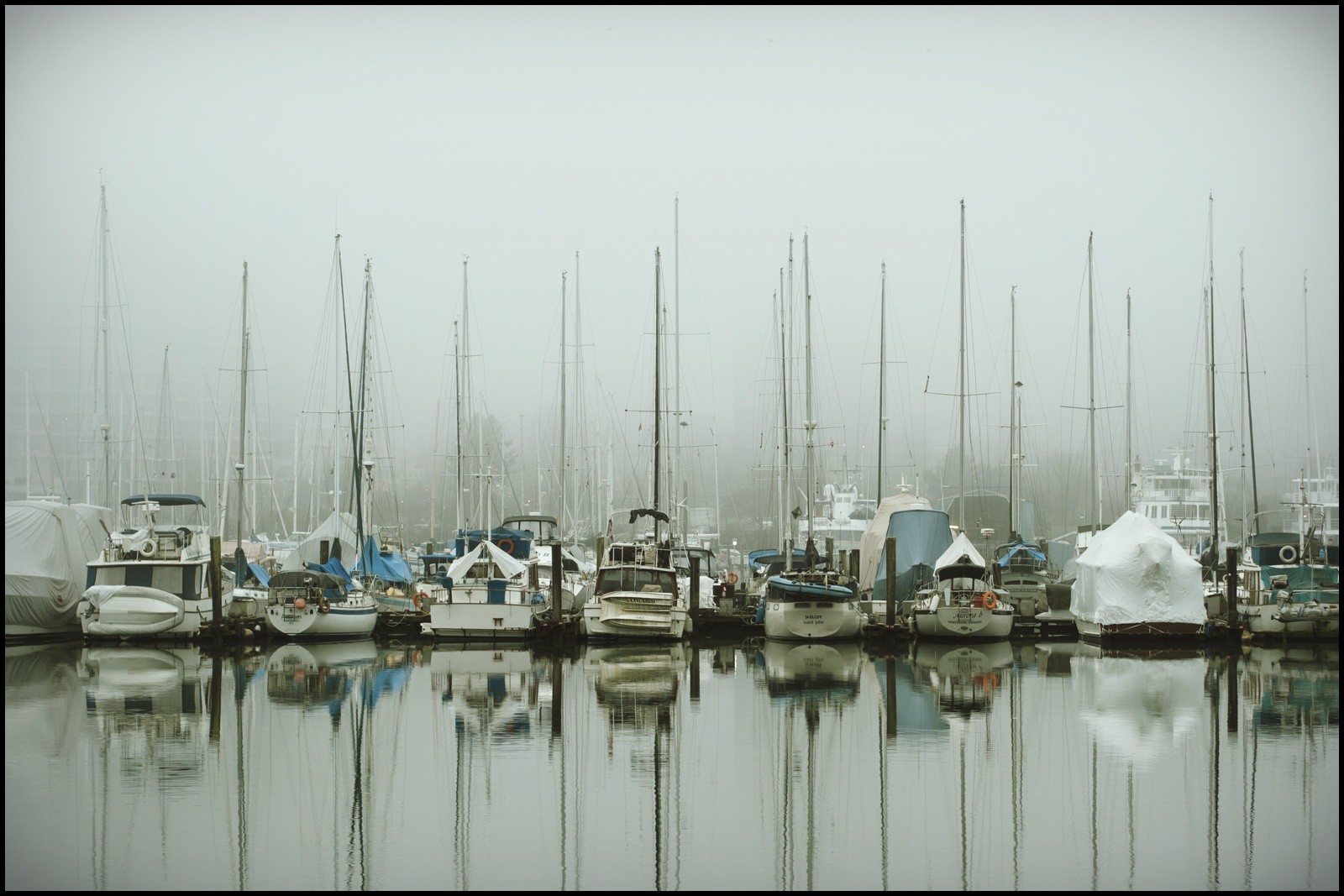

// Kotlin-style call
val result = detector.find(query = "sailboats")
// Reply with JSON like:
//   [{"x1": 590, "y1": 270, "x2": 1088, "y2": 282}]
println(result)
[{"x1": 5, "y1": 182, "x2": 1342, "y2": 640}]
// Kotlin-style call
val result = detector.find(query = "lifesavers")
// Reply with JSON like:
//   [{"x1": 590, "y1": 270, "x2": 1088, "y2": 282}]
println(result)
[
  {"x1": 414, "y1": 592, "x2": 430, "y2": 607},
  {"x1": 497, "y1": 538, "x2": 514, "y2": 555},
  {"x1": 727, "y1": 573, "x2": 739, "y2": 585},
  {"x1": 320, "y1": 596, "x2": 330, "y2": 613},
  {"x1": 1279, "y1": 544, "x2": 1298, "y2": 564},
  {"x1": 983, "y1": 591, "x2": 997, "y2": 609},
  {"x1": 138, "y1": 538, "x2": 156, "y2": 558}
]
